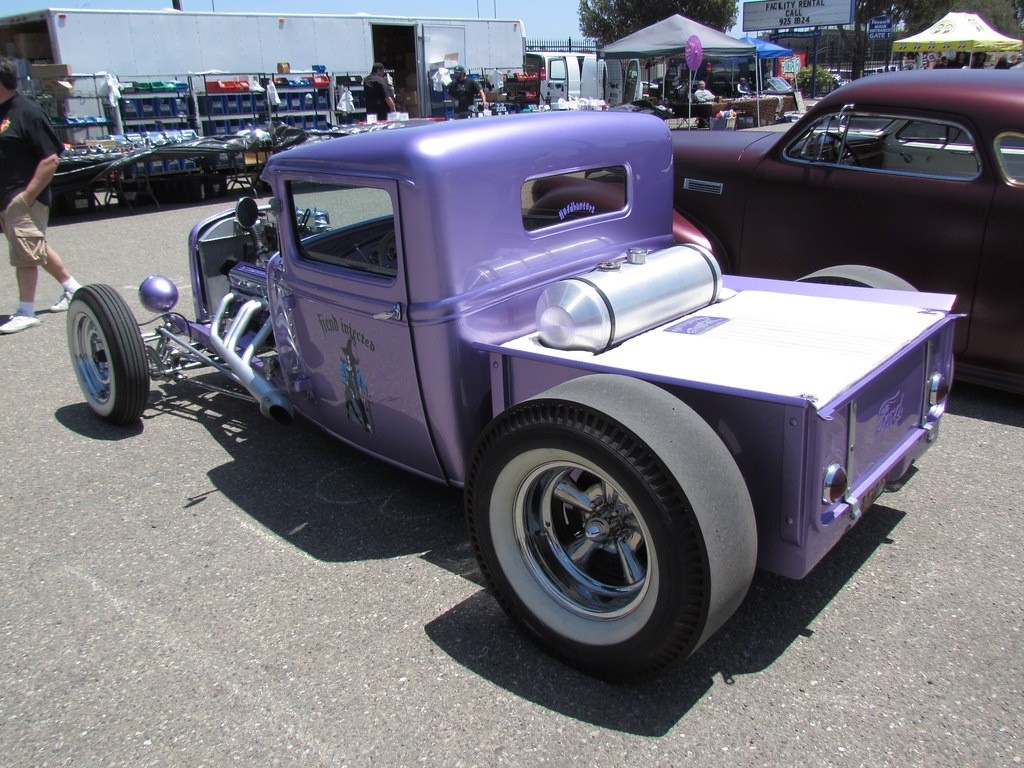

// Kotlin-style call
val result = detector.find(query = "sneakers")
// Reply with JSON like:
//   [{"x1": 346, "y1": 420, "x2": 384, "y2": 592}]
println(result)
[
  {"x1": 49, "y1": 292, "x2": 72, "y2": 312},
  {"x1": 0, "y1": 309, "x2": 39, "y2": 332}
]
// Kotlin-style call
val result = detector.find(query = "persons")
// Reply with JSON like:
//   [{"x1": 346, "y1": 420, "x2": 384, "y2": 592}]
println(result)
[
  {"x1": 0, "y1": 58, "x2": 83, "y2": 334},
  {"x1": 363, "y1": 62, "x2": 397, "y2": 120},
  {"x1": 695, "y1": 81, "x2": 716, "y2": 102},
  {"x1": 737, "y1": 78, "x2": 752, "y2": 95},
  {"x1": 449, "y1": 66, "x2": 486, "y2": 113},
  {"x1": 925, "y1": 50, "x2": 1023, "y2": 70}
]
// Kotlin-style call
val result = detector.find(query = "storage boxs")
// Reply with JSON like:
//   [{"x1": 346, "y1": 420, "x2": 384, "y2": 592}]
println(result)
[{"x1": 31, "y1": 62, "x2": 540, "y2": 178}]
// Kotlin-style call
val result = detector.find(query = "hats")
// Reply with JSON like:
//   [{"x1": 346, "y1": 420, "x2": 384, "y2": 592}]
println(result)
[{"x1": 454, "y1": 66, "x2": 464, "y2": 73}]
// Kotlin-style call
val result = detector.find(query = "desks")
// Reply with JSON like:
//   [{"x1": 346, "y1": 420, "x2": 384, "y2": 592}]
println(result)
[{"x1": 673, "y1": 93, "x2": 798, "y2": 131}]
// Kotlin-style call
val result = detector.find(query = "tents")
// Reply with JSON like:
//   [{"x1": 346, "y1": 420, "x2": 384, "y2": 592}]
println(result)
[
  {"x1": 671, "y1": 35, "x2": 797, "y2": 94},
  {"x1": 595, "y1": 13, "x2": 760, "y2": 129},
  {"x1": 889, "y1": 11, "x2": 1023, "y2": 71}
]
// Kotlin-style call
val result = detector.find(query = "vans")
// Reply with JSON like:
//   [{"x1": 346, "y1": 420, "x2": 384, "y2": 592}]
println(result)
[{"x1": 526, "y1": 52, "x2": 643, "y2": 106}]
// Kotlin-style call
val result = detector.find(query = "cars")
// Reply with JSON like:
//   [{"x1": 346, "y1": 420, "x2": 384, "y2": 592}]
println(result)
[
  {"x1": 524, "y1": 69, "x2": 1024, "y2": 396},
  {"x1": 64, "y1": 108, "x2": 972, "y2": 682},
  {"x1": 640, "y1": 69, "x2": 738, "y2": 95}
]
